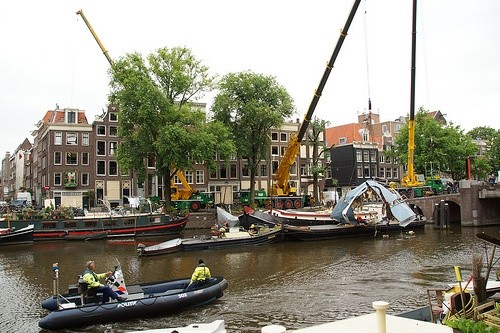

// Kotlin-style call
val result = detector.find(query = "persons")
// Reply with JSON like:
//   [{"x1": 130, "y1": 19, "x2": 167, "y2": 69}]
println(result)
[
  {"x1": 488, "y1": 174, "x2": 495, "y2": 190},
  {"x1": 445, "y1": 180, "x2": 460, "y2": 194},
  {"x1": 189, "y1": 259, "x2": 211, "y2": 283},
  {"x1": 82, "y1": 261, "x2": 127, "y2": 303},
  {"x1": 211, "y1": 220, "x2": 259, "y2": 238}
]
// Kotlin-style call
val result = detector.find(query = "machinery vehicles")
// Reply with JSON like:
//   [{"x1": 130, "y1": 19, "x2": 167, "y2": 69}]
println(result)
[
  {"x1": 76, "y1": 9, "x2": 207, "y2": 212},
  {"x1": 391, "y1": 0, "x2": 432, "y2": 200},
  {"x1": 251, "y1": 0, "x2": 378, "y2": 208}
]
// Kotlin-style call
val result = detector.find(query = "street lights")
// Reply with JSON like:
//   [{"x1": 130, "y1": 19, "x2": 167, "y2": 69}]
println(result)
[
  {"x1": 494, "y1": 170, "x2": 498, "y2": 182},
  {"x1": 123, "y1": 196, "x2": 133, "y2": 215},
  {"x1": 98, "y1": 198, "x2": 111, "y2": 217},
  {"x1": 139, "y1": 196, "x2": 152, "y2": 215},
  {"x1": 465, "y1": 156, "x2": 477, "y2": 180}
]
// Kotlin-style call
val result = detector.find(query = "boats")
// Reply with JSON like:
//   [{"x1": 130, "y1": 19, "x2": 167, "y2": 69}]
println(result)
[
  {"x1": 0, "y1": 224, "x2": 34, "y2": 244},
  {"x1": 38, "y1": 257, "x2": 229, "y2": 331},
  {"x1": 136, "y1": 238, "x2": 181, "y2": 254},
  {"x1": 240, "y1": 204, "x2": 426, "y2": 232},
  {"x1": 33, "y1": 228, "x2": 111, "y2": 241},
  {"x1": 181, "y1": 222, "x2": 283, "y2": 249},
  {"x1": 95, "y1": 217, "x2": 187, "y2": 236}
]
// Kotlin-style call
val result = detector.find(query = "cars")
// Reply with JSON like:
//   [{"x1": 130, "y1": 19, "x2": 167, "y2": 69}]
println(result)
[
  {"x1": 442, "y1": 178, "x2": 456, "y2": 187},
  {"x1": 32, "y1": 204, "x2": 42, "y2": 210},
  {"x1": 0, "y1": 201, "x2": 7, "y2": 208}
]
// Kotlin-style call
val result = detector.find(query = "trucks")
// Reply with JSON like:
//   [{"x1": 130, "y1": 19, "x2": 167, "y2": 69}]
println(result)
[{"x1": 17, "y1": 192, "x2": 32, "y2": 208}]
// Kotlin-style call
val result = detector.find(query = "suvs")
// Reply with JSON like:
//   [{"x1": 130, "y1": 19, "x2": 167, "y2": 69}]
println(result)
[{"x1": 10, "y1": 200, "x2": 27, "y2": 212}]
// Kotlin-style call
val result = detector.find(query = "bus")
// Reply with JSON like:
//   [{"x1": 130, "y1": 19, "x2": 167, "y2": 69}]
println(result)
[{"x1": 201, "y1": 192, "x2": 266, "y2": 208}]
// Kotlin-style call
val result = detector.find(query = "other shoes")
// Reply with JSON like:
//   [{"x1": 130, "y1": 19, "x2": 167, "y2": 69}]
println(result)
[{"x1": 118, "y1": 297, "x2": 127, "y2": 303}]
